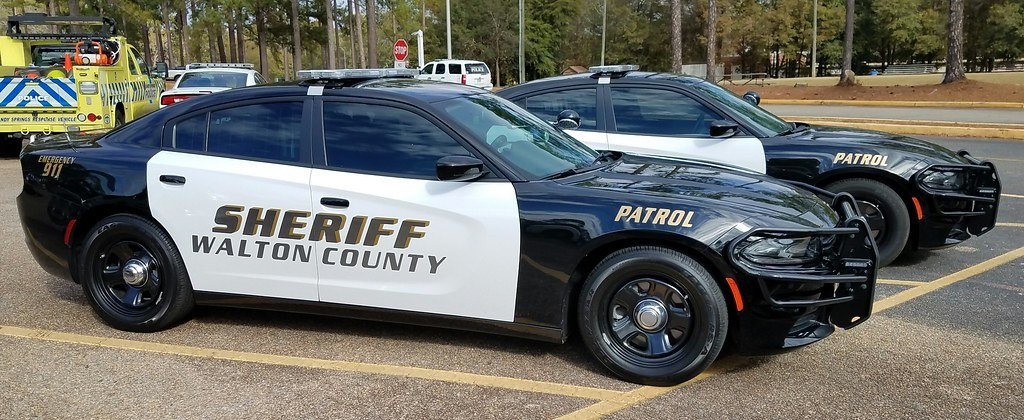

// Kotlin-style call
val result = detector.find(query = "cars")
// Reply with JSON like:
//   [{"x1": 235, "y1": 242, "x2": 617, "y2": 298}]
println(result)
[
  {"x1": 15, "y1": 69, "x2": 881, "y2": 387},
  {"x1": 151, "y1": 63, "x2": 269, "y2": 109},
  {"x1": 494, "y1": 65, "x2": 1003, "y2": 266}
]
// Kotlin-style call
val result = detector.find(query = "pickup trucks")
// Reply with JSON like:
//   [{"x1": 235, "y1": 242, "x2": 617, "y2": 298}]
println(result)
[{"x1": 0, "y1": 13, "x2": 168, "y2": 160}]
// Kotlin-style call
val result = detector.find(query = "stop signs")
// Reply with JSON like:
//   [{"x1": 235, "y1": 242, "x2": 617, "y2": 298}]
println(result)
[{"x1": 393, "y1": 39, "x2": 408, "y2": 61}]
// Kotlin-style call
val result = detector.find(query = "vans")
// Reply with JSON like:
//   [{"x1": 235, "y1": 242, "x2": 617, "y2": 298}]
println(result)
[{"x1": 414, "y1": 59, "x2": 493, "y2": 92}]
